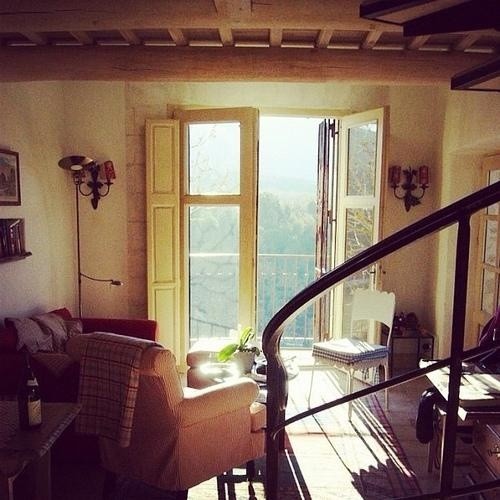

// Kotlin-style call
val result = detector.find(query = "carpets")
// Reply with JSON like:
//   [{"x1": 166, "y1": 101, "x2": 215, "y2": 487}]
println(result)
[{"x1": 193, "y1": 351, "x2": 424, "y2": 500}]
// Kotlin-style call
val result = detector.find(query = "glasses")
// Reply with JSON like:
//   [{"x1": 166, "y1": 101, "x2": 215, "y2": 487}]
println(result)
[
  {"x1": 388, "y1": 164, "x2": 430, "y2": 212},
  {"x1": 73, "y1": 160, "x2": 117, "y2": 210}
]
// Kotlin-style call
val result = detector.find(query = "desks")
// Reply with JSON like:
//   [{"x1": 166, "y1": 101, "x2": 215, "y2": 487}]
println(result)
[{"x1": 2, "y1": 400, "x2": 85, "y2": 500}]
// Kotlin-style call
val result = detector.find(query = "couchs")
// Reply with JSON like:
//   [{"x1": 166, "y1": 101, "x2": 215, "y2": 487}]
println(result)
[
  {"x1": 76, "y1": 332, "x2": 266, "y2": 499},
  {"x1": 186, "y1": 337, "x2": 240, "y2": 391},
  {"x1": 1, "y1": 307, "x2": 158, "y2": 407}
]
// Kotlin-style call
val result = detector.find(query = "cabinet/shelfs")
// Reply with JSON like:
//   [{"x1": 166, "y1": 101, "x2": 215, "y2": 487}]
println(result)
[{"x1": 18, "y1": 355, "x2": 44, "y2": 429}]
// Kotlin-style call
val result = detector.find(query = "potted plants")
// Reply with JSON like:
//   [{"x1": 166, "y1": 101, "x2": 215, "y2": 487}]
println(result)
[
  {"x1": 218, "y1": 325, "x2": 259, "y2": 377},
  {"x1": 0, "y1": 148, "x2": 22, "y2": 206}
]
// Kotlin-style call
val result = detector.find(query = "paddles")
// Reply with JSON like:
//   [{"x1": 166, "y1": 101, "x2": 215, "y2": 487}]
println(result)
[{"x1": 193, "y1": 351, "x2": 424, "y2": 500}]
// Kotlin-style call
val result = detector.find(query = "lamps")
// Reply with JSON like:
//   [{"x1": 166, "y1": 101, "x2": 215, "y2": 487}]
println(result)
[
  {"x1": 306, "y1": 287, "x2": 396, "y2": 422},
  {"x1": 73, "y1": 160, "x2": 117, "y2": 210},
  {"x1": 388, "y1": 164, "x2": 430, "y2": 212}
]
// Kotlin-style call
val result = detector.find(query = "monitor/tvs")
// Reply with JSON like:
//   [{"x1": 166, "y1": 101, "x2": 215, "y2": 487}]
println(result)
[
  {"x1": 0, "y1": 218, "x2": 23, "y2": 258},
  {"x1": 463, "y1": 374, "x2": 500, "y2": 397}
]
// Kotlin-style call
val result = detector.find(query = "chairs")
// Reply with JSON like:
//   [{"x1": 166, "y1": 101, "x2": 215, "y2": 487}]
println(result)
[{"x1": 306, "y1": 287, "x2": 396, "y2": 422}]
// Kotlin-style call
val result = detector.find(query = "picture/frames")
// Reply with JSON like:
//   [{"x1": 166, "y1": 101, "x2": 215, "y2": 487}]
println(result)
[{"x1": 0, "y1": 148, "x2": 22, "y2": 206}]
